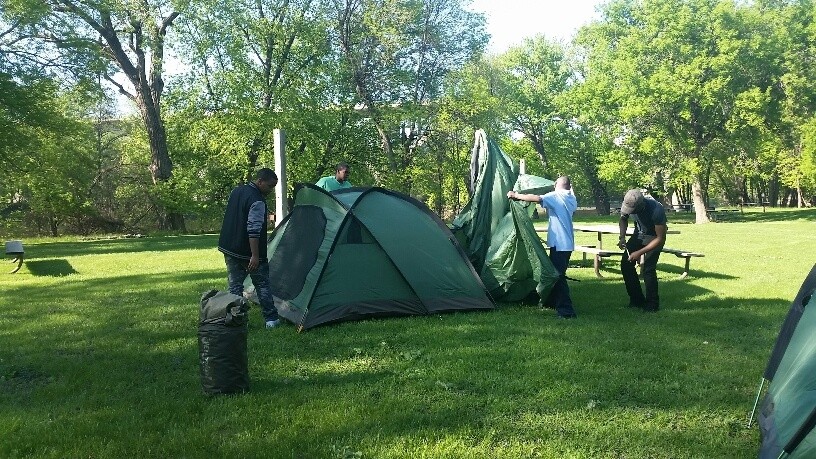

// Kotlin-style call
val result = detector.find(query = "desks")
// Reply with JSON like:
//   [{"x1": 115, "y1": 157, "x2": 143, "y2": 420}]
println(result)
[
  {"x1": 707, "y1": 210, "x2": 718, "y2": 221},
  {"x1": 670, "y1": 204, "x2": 694, "y2": 213},
  {"x1": 534, "y1": 227, "x2": 681, "y2": 267},
  {"x1": 739, "y1": 202, "x2": 771, "y2": 213},
  {"x1": 721, "y1": 210, "x2": 739, "y2": 221}
]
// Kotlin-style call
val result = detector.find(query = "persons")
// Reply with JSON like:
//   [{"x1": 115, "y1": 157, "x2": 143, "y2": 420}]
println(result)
[
  {"x1": 506, "y1": 176, "x2": 577, "y2": 319},
  {"x1": 616, "y1": 187, "x2": 667, "y2": 314},
  {"x1": 314, "y1": 163, "x2": 353, "y2": 192},
  {"x1": 218, "y1": 168, "x2": 282, "y2": 329}
]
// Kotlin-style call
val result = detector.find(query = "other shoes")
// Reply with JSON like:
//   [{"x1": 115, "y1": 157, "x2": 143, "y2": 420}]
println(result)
[
  {"x1": 629, "y1": 300, "x2": 643, "y2": 308},
  {"x1": 645, "y1": 301, "x2": 659, "y2": 312}
]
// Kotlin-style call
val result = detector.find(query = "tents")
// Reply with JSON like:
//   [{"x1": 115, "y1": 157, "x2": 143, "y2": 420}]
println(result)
[
  {"x1": 240, "y1": 183, "x2": 499, "y2": 333},
  {"x1": 748, "y1": 262, "x2": 816, "y2": 459}
]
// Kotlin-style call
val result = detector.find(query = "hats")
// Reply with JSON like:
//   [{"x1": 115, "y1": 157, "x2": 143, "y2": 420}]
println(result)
[{"x1": 620, "y1": 190, "x2": 644, "y2": 215}]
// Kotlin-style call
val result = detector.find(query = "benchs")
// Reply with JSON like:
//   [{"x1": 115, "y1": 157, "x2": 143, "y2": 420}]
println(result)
[
  {"x1": 667, "y1": 208, "x2": 689, "y2": 213},
  {"x1": 717, "y1": 214, "x2": 733, "y2": 220},
  {"x1": 5, "y1": 241, "x2": 24, "y2": 274},
  {"x1": 574, "y1": 246, "x2": 705, "y2": 280}
]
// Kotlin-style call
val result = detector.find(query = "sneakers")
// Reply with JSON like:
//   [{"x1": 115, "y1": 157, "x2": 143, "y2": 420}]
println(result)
[{"x1": 266, "y1": 319, "x2": 281, "y2": 329}]
